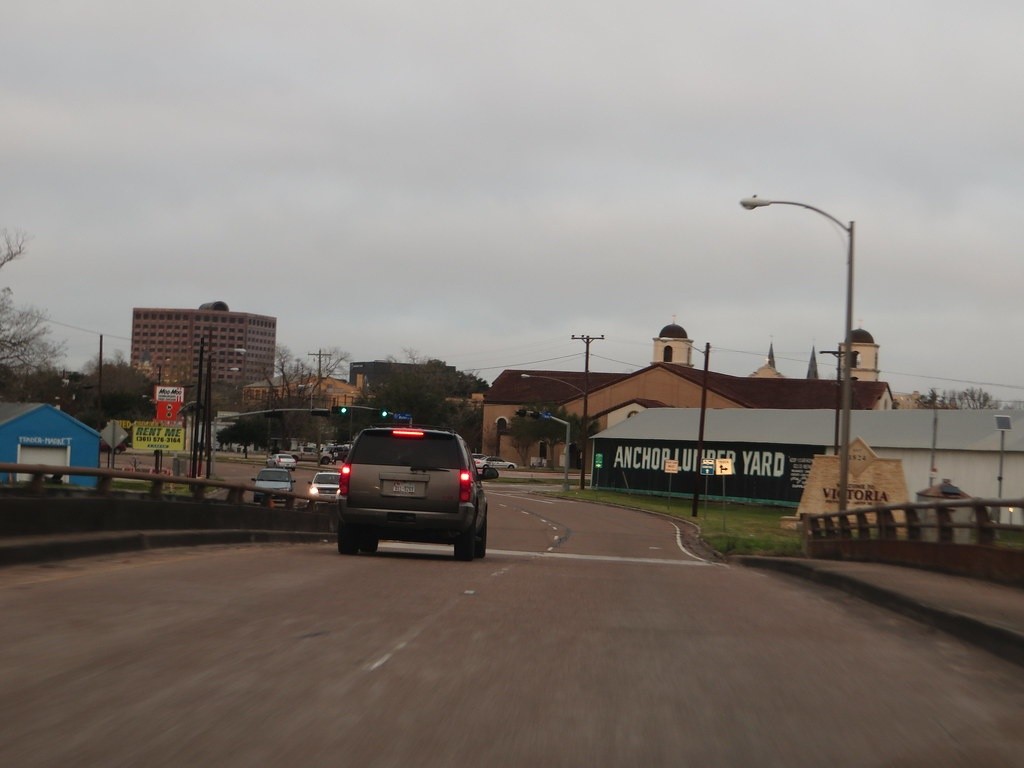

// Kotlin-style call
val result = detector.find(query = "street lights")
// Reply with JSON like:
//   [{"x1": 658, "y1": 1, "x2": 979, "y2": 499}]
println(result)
[
  {"x1": 993, "y1": 414, "x2": 1012, "y2": 524},
  {"x1": 739, "y1": 197, "x2": 856, "y2": 565},
  {"x1": 192, "y1": 337, "x2": 247, "y2": 479},
  {"x1": 520, "y1": 373, "x2": 588, "y2": 490},
  {"x1": 658, "y1": 336, "x2": 710, "y2": 517}
]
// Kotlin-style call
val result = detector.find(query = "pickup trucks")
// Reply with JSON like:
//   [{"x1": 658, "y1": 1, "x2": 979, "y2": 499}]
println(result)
[{"x1": 279, "y1": 446, "x2": 333, "y2": 465}]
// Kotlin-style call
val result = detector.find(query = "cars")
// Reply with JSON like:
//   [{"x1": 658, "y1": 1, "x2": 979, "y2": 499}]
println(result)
[
  {"x1": 251, "y1": 468, "x2": 296, "y2": 508},
  {"x1": 100, "y1": 435, "x2": 126, "y2": 455},
  {"x1": 475, "y1": 456, "x2": 518, "y2": 470},
  {"x1": 472, "y1": 453, "x2": 487, "y2": 462},
  {"x1": 264, "y1": 453, "x2": 296, "y2": 471},
  {"x1": 307, "y1": 471, "x2": 342, "y2": 503}
]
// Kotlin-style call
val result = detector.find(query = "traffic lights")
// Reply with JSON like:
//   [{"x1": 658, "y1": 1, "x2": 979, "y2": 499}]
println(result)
[
  {"x1": 332, "y1": 406, "x2": 349, "y2": 414},
  {"x1": 379, "y1": 409, "x2": 389, "y2": 419}
]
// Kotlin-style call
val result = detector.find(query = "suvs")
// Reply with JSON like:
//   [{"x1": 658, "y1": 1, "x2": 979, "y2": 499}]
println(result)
[
  {"x1": 333, "y1": 423, "x2": 499, "y2": 562},
  {"x1": 323, "y1": 444, "x2": 353, "y2": 463}
]
